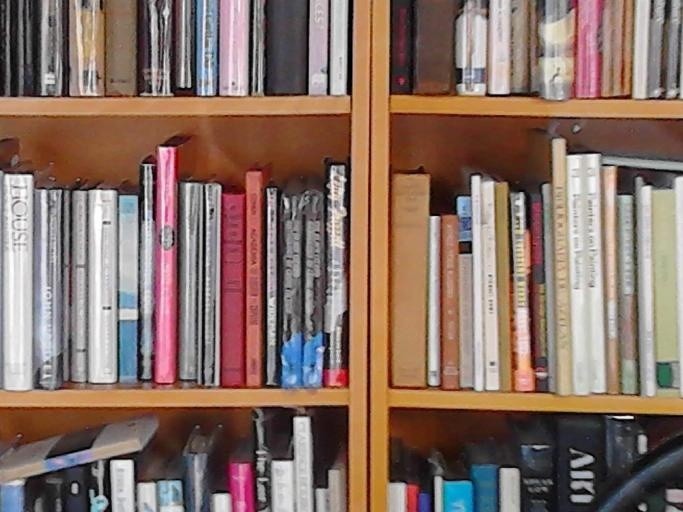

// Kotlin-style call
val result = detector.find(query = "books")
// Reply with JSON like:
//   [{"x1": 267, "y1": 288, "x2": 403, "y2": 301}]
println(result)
[
  {"x1": 388, "y1": 413, "x2": 683, "y2": 512},
  {"x1": 392, "y1": 1, "x2": 683, "y2": 101}
]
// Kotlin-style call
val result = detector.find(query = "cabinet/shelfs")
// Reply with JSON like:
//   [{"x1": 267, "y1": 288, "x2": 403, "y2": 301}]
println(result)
[{"x1": 0, "y1": 0, "x2": 682, "y2": 511}]
